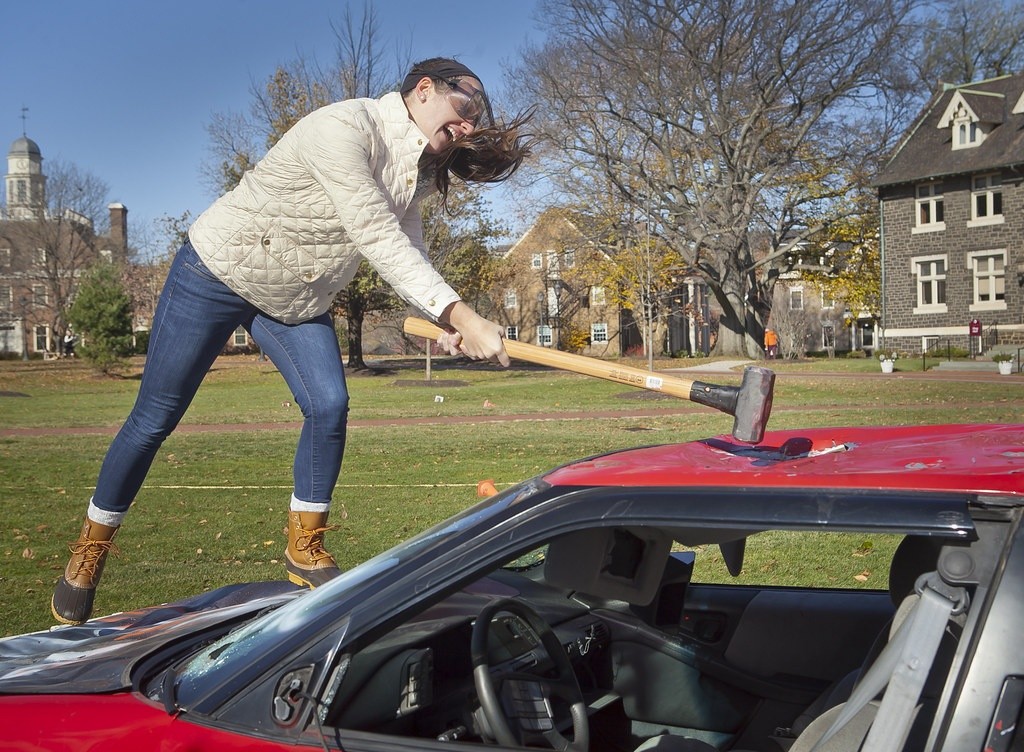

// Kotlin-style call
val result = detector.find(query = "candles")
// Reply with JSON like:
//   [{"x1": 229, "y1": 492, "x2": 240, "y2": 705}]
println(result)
[{"x1": 877, "y1": 348, "x2": 900, "y2": 373}]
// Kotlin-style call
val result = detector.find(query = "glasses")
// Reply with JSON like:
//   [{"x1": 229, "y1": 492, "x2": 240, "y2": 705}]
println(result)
[{"x1": 445, "y1": 77, "x2": 492, "y2": 127}]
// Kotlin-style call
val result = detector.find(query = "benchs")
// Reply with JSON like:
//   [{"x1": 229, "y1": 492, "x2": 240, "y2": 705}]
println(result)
[{"x1": 43, "y1": 352, "x2": 75, "y2": 361}]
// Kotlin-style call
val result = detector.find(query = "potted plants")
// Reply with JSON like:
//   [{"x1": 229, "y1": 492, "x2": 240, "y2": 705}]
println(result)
[{"x1": 991, "y1": 352, "x2": 1017, "y2": 375}]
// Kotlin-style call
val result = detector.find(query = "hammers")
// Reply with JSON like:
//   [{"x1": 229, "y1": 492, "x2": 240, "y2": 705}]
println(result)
[{"x1": 402, "y1": 314, "x2": 776, "y2": 444}]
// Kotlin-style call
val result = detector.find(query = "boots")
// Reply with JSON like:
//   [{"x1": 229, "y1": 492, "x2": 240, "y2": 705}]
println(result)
[
  {"x1": 284, "y1": 506, "x2": 345, "y2": 591},
  {"x1": 52, "y1": 516, "x2": 120, "y2": 626}
]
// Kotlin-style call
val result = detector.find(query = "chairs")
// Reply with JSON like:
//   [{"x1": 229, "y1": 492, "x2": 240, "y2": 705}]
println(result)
[{"x1": 716, "y1": 520, "x2": 976, "y2": 752}]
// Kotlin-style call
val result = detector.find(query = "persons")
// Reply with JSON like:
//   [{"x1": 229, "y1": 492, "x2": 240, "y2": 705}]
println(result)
[
  {"x1": 64, "y1": 330, "x2": 74, "y2": 358},
  {"x1": 764, "y1": 329, "x2": 779, "y2": 359},
  {"x1": 52, "y1": 57, "x2": 532, "y2": 625}
]
